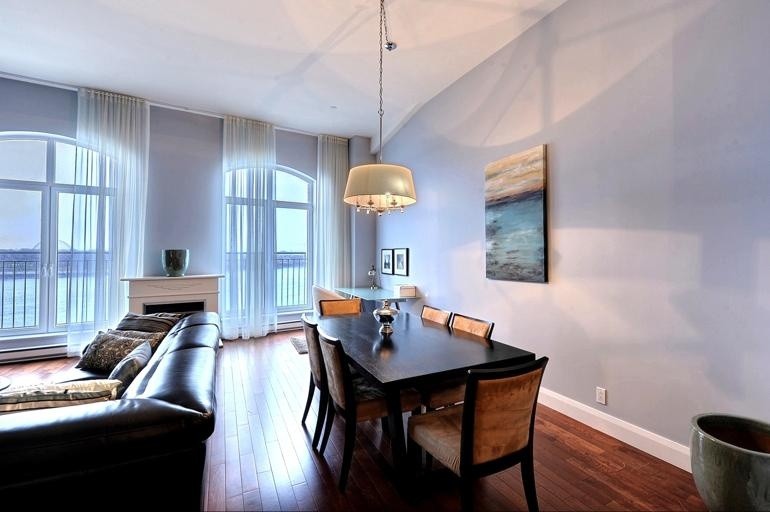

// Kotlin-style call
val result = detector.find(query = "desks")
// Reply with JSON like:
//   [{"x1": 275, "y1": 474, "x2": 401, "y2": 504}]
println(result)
[{"x1": 334, "y1": 287, "x2": 421, "y2": 310}]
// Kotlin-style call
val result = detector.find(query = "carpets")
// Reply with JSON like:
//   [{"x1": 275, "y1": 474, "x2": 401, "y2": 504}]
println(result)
[{"x1": 289, "y1": 336, "x2": 308, "y2": 354}]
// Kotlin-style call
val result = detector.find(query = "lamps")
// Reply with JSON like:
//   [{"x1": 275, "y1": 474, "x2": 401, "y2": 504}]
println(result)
[{"x1": 343, "y1": 0, "x2": 417, "y2": 216}]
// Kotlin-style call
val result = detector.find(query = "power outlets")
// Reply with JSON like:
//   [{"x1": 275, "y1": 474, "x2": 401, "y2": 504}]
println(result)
[{"x1": 595, "y1": 386, "x2": 607, "y2": 405}]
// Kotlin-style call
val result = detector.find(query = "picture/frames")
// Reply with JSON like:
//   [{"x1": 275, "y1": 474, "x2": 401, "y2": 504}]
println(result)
[
  {"x1": 380, "y1": 249, "x2": 394, "y2": 275},
  {"x1": 393, "y1": 248, "x2": 408, "y2": 276}
]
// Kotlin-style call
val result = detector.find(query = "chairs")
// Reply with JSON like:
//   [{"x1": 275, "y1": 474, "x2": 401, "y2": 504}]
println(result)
[
  {"x1": 421, "y1": 313, "x2": 494, "y2": 411},
  {"x1": 311, "y1": 283, "x2": 347, "y2": 318},
  {"x1": 318, "y1": 332, "x2": 421, "y2": 493},
  {"x1": 318, "y1": 297, "x2": 362, "y2": 317},
  {"x1": 406, "y1": 356, "x2": 549, "y2": 512},
  {"x1": 301, "y1": 312, "x2": 362, "y2": 449},
  {"x1": 420, "y1": 305, "x2": 452, "y2": 326}
]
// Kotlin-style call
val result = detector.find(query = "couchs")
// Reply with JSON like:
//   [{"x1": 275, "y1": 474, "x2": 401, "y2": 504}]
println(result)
[{"x1": 0, "y1": 311, "x2": 221, "y2": 512}]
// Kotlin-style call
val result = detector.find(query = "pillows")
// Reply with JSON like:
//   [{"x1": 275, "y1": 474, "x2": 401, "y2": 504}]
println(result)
[
  {"x1": 115, "y1": 312, "x2": 186, "y2": 333},
  {"x1": 108, "y1": 339, "x2": 153, "y2": 398},
  {"x1": 74, "y1": 329, "x2": 167, "y2": 376},
  {"x1": 0, "y1": 378, "x2": 124, "y2": 404}
]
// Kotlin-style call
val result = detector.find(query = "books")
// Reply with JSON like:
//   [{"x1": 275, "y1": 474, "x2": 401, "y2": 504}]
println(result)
[{"x1": 400, "y1": 285, "x2": 416, "y2": 297}]
